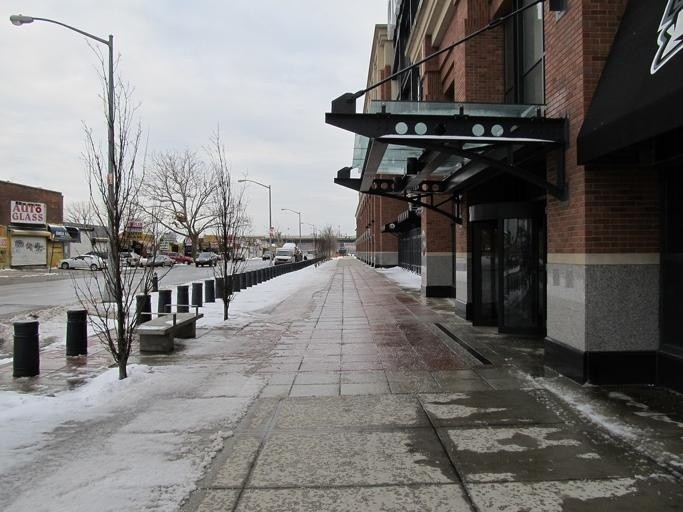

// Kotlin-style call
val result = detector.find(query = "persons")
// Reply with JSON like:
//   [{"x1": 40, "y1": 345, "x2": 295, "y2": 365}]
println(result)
[{"x1": 294, "y1": 250, "x2": 307, "y2": 262}]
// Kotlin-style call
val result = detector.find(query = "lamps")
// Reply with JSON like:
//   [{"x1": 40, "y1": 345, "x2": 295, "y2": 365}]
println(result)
[{"x1": 406, "y1": 156, "x2": 426, "y2": 176}]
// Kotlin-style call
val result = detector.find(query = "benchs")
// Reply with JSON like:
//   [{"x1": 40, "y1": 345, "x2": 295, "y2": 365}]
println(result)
[{"x1": 135, "y1": 304, "x2": 204, "y2": 351}]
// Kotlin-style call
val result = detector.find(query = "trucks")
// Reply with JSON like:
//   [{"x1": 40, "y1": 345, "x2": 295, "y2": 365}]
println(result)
[{"x1": 272, "y1": 248, "x2": 295, "y2": 266}]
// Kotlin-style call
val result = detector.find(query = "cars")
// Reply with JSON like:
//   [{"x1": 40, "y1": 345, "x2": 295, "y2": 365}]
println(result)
[
  {"x1": 262, "y1": 251, "x2": 274, "y2": 261},
  {"x1": 195, "y1": 252, "x2": 218, "y2": 266},
  {"x1": 120, "y1": 252, "x2": 194, "y2": 267},
  {"x1": 58, "y1": 255, "x2": 105, "y2": 271},
  {"x1": 294, "y1": 254, "x2": 307, "y2": 262},
  {"x1": 234, "y1": 253, "x2": 245, "y2": 262}
]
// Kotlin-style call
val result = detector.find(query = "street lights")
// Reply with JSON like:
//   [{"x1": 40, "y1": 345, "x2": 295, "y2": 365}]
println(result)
[
  {"x1": 238, "y1": 180, "x2": 271, "y2": 247},
  {"x1": 301, "y1": 223, "x2": 314, "y2": 249},
  {"x1": 282, "y1": 209, "x2": 301, "y2": 248},
  {"x1": 10, "y1": 15, "x2": 117, "y2": 302}
]
